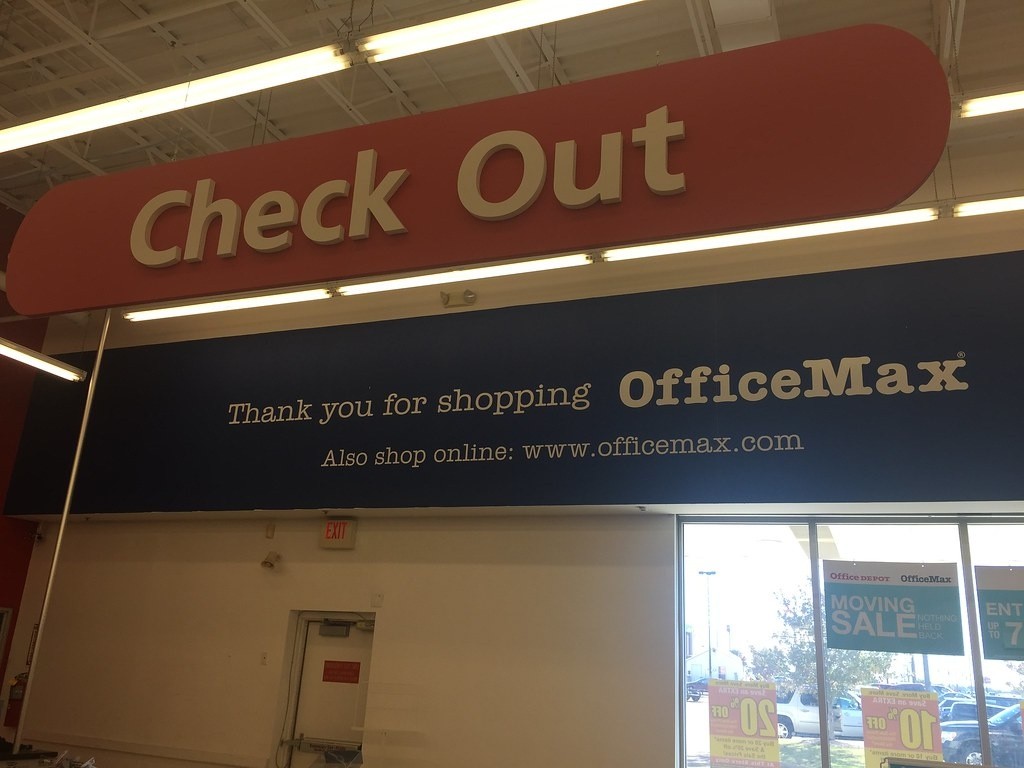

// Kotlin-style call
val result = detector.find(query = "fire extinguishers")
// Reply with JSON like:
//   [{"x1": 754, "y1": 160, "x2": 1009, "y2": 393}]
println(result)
[{"x1": 2, "y1": 673, "x2": 29, "y2": 728}]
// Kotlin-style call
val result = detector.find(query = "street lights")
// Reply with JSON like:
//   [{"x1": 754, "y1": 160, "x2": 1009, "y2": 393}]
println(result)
[{"x1": 699, "y1": 571, "x2": 716, "y2": 678}]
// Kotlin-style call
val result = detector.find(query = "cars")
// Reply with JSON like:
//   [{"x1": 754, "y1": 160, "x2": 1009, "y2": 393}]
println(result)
[
  {"x1": 851, "y1": 683, "x2": 925, "y2": 698},
  {"x1": 686, "y1": 678, "x2": 722, "y2": 692},
  {"x1": 777, "y1": 684, "x2": 864, "y2": 740},
  {"x1": 939, "y1": 703, "x2": 1024, "y2": 768},
  {"x1": 774, "y1": 676, "x2": 790, "y2": 682},
  {"x1": 930, "y1": 685, "x2": 1024, "y2": 721}
]
